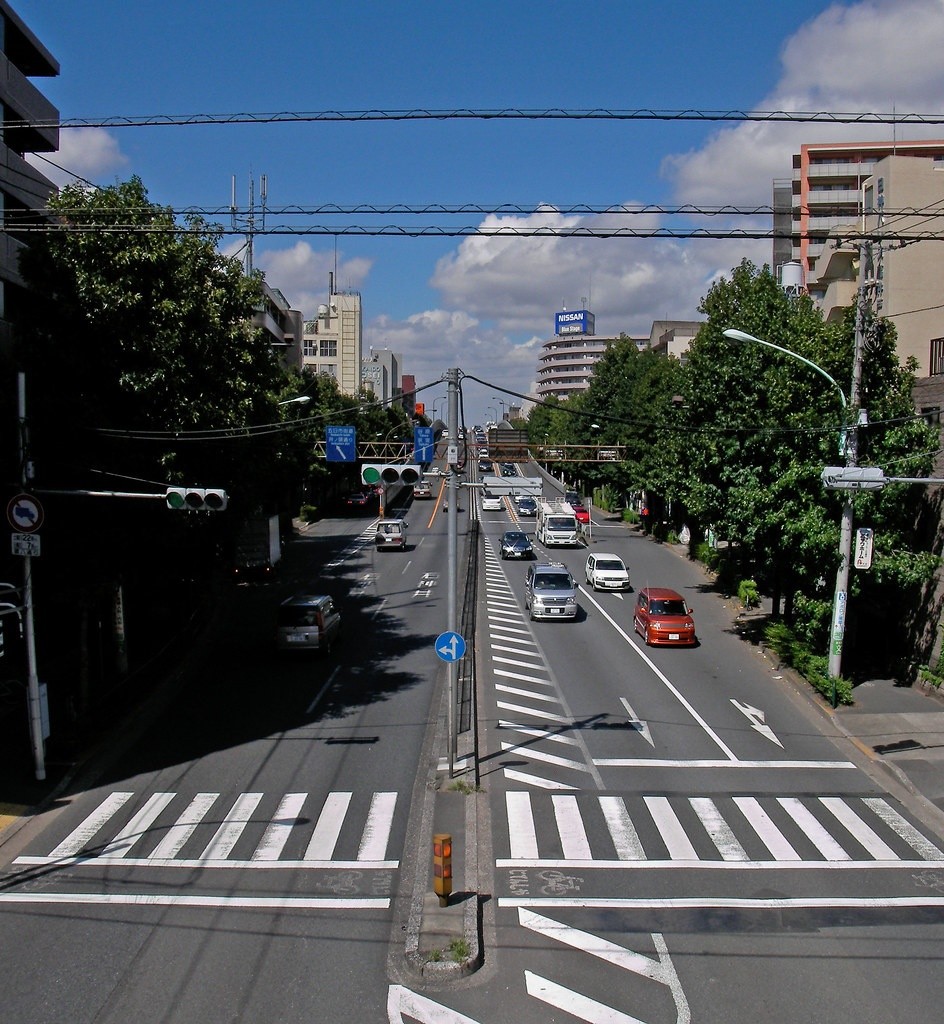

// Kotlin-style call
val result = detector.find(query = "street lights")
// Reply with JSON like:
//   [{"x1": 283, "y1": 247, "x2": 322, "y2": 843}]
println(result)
[
  {"x1": 492, "y1": 397, "x2": 504, "y2": 421},
  {"x1": 485, "y1": 413, "x2": 492, "y2": 422},
  {"x1": 379, "y1": 419, "x2": 420, "y2": 514},
  {"x1": 488, "y1": 407, "x2": 497, "y2": 423},
  {"x1": 433, "y1": 396, "x2": 446, "y2": 423},
  {"x1": 723, "y1": 327, "x2": 856, "y2": 679}
]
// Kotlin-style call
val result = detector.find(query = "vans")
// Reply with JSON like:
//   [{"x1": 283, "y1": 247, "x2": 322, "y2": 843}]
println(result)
[
  {"x1": 585, "y1": 552, "x2": 630, "y2": 591},
  {"x1": 441, "y1": 430, "x2": 449, "y2": 437},
  {"x1": 632, "y1": 587, "x2": 696, "y2": 647},
  {"x1": 275, "y1": 594, "x2": 342, "y2": 656},
  {"x1": 444, "y1": 494, "x2": 462, "y2": 511},
  {"x1": 413, "y1": 480, "x2": 434, "y2": 498},
  {"x1": 481, "y1": 489, "x2": 500, "y2": 510},
  {"x1": 376, "y1": 519, "x2": 409, "y2": 552},
  {"x1": 514, "y1": 495, "x2": 531, "y2": 503}
]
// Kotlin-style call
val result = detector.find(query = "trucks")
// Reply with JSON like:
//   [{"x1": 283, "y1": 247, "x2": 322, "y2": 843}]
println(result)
[
  {"x1": 526, "y1": 562, "x2": 579, "y2": 621},
  {"x1": 536, "y1": 497, "x2": 579, "y2": 549}
]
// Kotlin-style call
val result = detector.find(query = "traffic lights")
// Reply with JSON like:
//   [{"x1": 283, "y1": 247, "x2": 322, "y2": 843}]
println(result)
[
  {"x1": 361, "y1": 464, "x2": 423, "y2": 486},
  {"x1": 822, "y1": 467, "x2": 883, "y2": 490},
  {"x1": 166, "y1": 487, "x2": 227, "y2": 511}
]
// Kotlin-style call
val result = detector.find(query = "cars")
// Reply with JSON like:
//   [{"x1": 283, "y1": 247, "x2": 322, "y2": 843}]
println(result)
[
  {"x1": 500, "y1": 462, "x2": 517, "y2": 477},
  {"x1": 346, "y1": 493, "x2": 367, "y2": 508},
  {"x1": 516, "y1": 499, "x2": 538, "y2": 516},
  {"x1": 432, "y1": 467, "x2": 439, "y2": 472},
  {"x1": 458, "y1": 426, "x2": 466, "y2": 439},
  {"x1": 472, "y1": 426, "x2": 493, "y2": 471},
  {"x1": 564, "y1": 487, "x2": 589, "y2": 523},
  {"x1": 498, "y1": 531, "x2": 534, "y2": 559}
]
